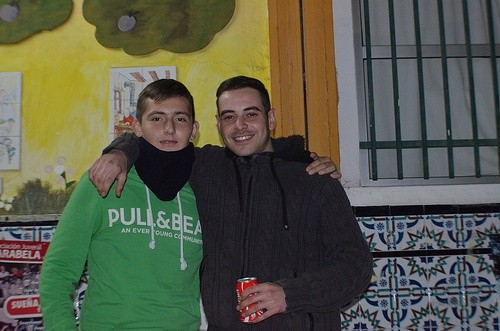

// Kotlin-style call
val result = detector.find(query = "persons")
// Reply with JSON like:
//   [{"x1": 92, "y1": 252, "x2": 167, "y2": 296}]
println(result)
[
  {"x1": 88, "y1": 76, "x2": 373, "y2": 331},
  {"x1": 36, "y1": 79, "x2": 341, "y2": 331},
  {"x1": 0, "y1": 264, "x2": 43, "y2": 331}
]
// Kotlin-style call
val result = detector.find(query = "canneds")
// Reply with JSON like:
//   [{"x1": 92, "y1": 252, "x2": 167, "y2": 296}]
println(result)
[{"x1": 236, "y1": 277, "x2": 264, "y2": 323}]
